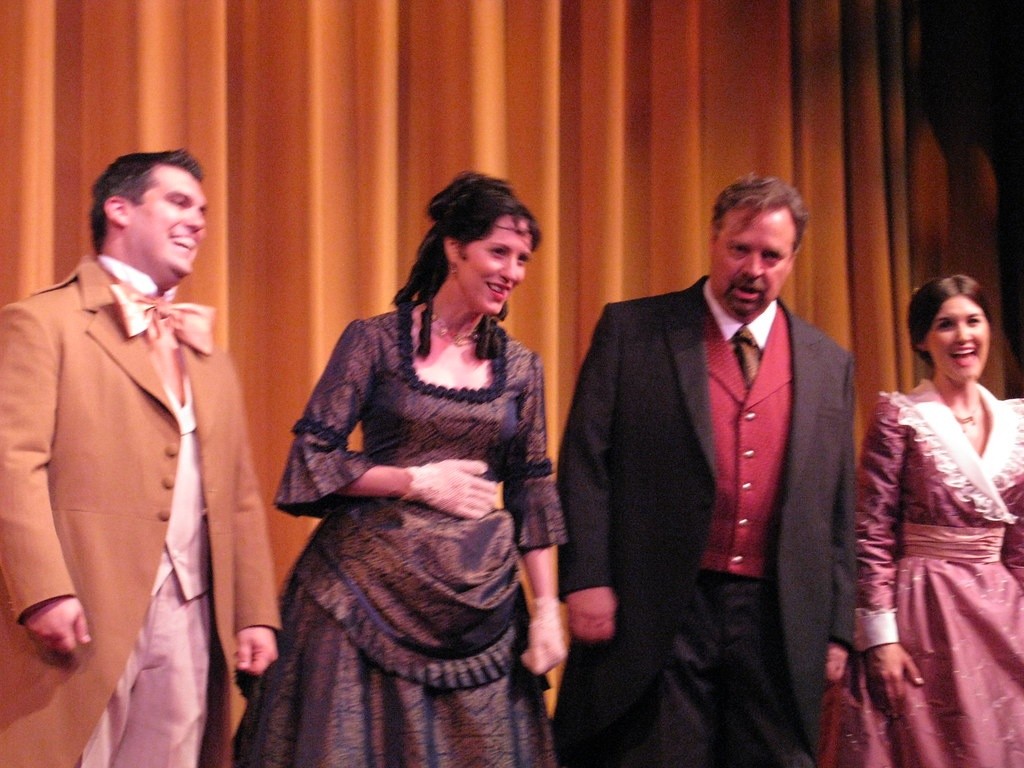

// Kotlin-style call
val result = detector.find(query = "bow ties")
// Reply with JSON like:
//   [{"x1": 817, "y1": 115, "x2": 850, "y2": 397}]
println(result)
[{"x1": 110, "y1": 283, "x2": 216, "y2": 354}]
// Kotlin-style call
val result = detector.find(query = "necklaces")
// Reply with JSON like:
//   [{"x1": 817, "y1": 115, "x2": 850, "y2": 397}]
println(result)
[{"x1": 432, "y1": 315, "x2": 480, "y2": 346}]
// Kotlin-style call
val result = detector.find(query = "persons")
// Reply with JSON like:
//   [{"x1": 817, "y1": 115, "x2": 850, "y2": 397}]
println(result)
[
  {"x1": 0, "y1": 148, "x2": 283, "y2": 768},
  {"x1": 836, "y1": 273, "x2": 1024, "y2": 768},
  {"x1": 557, "y1": 175, "x2": 859, "y2": 768},
  {"x1": 230, "y1": 172, "x2": 569, "y2": 768}
]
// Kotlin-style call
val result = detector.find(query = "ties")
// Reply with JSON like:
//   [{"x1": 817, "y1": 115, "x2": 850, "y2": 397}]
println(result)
[{"x1": 737, "y1": 327, "x2": 759, "y2": 386}]
